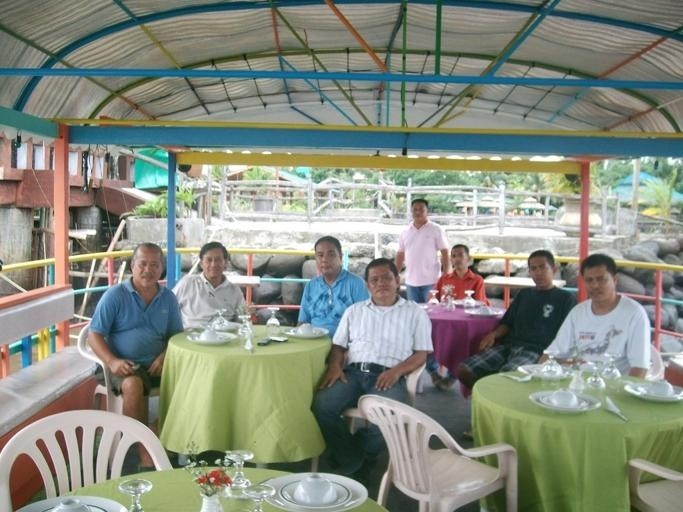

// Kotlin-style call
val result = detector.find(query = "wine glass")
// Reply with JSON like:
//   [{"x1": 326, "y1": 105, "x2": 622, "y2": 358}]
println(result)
[
  {"x1": 262, "y1": 305, "x2": 282, "y2": 333},
  {"x1": 234, "y1": 313, "x2": 254, "y2": 335},
  {"x1": 118, "y1": 478, "x2": 151, "y2": 512},
  {"x1": 219, "y1": 446, "x2": 254, "y2": 500},
  {"x1": 210, "y1": 307, "x2": 229, "y2": 329},
  {"x1": 428, "y1": 286, "x2": 477, "y2": 310},
  {"x1": 241, "y1": 482, "x2": 275, "y2": 511}
]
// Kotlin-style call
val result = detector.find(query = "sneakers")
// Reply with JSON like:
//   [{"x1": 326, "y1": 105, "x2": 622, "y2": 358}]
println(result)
[{"x1": 429, "y1": 369, "x2": 455, "y2": 392}]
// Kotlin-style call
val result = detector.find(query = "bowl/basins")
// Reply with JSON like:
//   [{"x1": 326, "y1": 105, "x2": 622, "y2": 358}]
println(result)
[
  {"x1": 289, "y1": 472, "x2": 338, "y2": 504},
  {"x1": 50, "y1": 498, "x2": 89, "y2": 512}
]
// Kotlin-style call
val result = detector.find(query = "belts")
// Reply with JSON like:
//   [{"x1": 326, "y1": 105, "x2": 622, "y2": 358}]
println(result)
[{"x1": 346, "y1": 361, "x2": 392, "y2": 375}]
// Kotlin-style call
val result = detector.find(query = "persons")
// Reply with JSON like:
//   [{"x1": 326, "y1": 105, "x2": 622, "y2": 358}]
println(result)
[
  {"x1": 424, "y1": 243, "x2": 493, "y2": 393},
  {"x1": 453, "y1": 249, "x2": 577, "y2": 438},
  {"x1": 537, "y1": 253, "x2": 651, "y2": 379},
  {"x1": 88, "y1": 241, "x2": 182, "y2": 472},
  {"x1": 395, "y1": 198, "x2": 449, "y2": 304},
  {"x1": 309, "y1": 257, "x2": 434, "y2": 486},
  {"x1": 174, "y1": 242, "x2": 244, "y2": 332},
  {"x1": 296, "y1": 236, "x2": 372, "y2": 339}
]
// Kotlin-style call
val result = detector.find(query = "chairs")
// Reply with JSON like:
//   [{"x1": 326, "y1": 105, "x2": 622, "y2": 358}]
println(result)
[
  {"x1": 310, "y1": 356, "x2": 429, "y2": 473},
  {"x1": 75, "y1": 323, "x2": 162, "y2": 472},
  {"x1": 0, "y1": 406, "x2": 175, "y2": 511},
  {"x1": 354, "y1": 392, "x2": 521, "y2": 512},
  {"x1": 649, "y1": 343, "x2": 666, "y2": 381}
]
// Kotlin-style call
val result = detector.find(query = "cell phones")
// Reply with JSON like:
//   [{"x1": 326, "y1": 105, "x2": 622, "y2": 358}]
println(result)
[
  {"x1": 257, "y1": 337, "x2": 271, "y2": 346},
  {"x1": 130, "y1": 363, "x2": 140, "y2": 369},
  {"x1": 271, "y1": 336, "x2": 288, "y2": 342}
]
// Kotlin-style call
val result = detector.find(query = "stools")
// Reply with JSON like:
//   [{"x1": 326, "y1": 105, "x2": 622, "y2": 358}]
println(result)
[{"x1": 622, "y1": 454, "x2": 682, "y2": 512}]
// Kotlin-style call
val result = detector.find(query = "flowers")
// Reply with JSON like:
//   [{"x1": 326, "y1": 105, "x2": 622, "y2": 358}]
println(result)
[{"x1": 178, "y1": 438, "x2": 236, "y2": 494}]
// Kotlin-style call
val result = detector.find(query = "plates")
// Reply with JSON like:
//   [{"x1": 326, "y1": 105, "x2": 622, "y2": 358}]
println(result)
[
  {"x1": 13, "y1": 495, "x2": 127, "y2": 512},
  {"x1": 260, "y1": 471, "x2": 368, "y2": 511},
  {"x1": 451, "y1": 298, "x2": 503, "y2": 317},
  {"x1": 188, "y1": 322, "x2": 331, "y2": 346},
  {"x1": 511, "y1": 348, "x2": 682, "y2": 412}
]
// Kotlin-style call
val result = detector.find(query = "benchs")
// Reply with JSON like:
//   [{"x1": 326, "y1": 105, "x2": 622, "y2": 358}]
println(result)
[{"x1": 0, "y1": 284, "x2": 108, "y2": 508}]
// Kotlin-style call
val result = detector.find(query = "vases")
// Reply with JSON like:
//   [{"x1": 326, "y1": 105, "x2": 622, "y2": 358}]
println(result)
[{"x1": 198, "y1": 491, "x2": 224, "y2": 512}]
